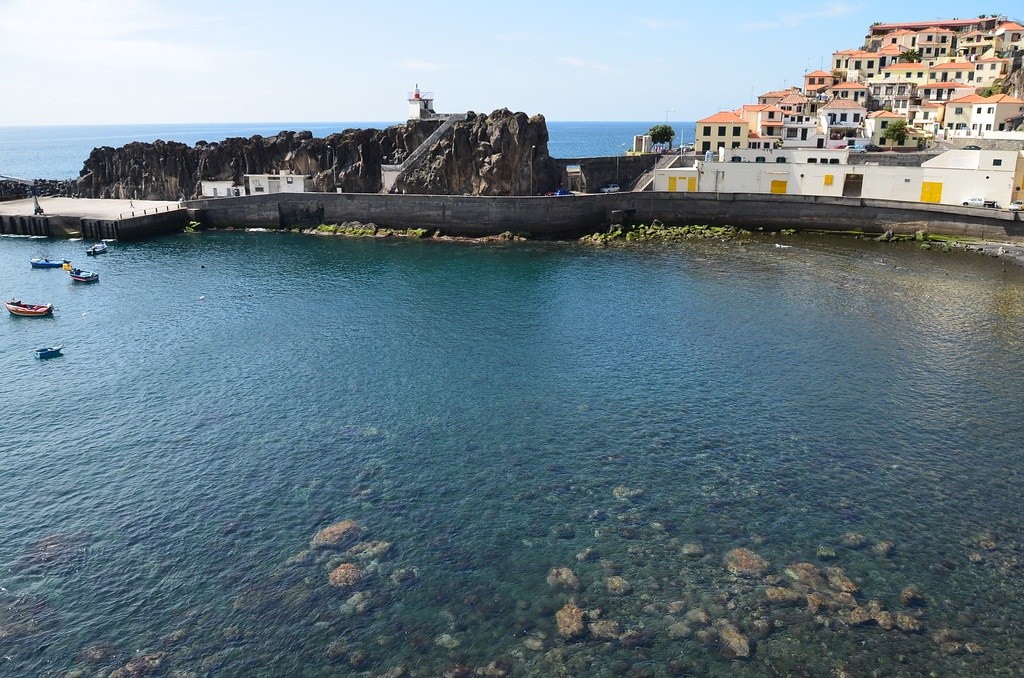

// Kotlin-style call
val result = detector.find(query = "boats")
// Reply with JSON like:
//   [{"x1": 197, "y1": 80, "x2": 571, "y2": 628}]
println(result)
[
  {"x1": 5, "y1": 300, "x2": 53, "y2": 317},
  {"x1": 34, "y1": 344, "x2": 64, "y2": 359},
  {"x1": 69, "y1": 267, "x2": 100, "y2": 282},
  {"x1": 29, "y1": 259, "x2": 71, "y2": 268},
  {"x1": 85, "y1": 242, "x2": 109, "y2": 256}
]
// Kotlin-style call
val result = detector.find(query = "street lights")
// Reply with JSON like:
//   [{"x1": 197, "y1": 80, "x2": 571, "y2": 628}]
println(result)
[
  {"x1": 616, "y1": 143, "x2": 626, "y2": 186},
  {"x1": 666, "y1": 108, "x2": 676, "y2": 126},
  {"x1": 530, "y1": 145, "x2": 536, "y2": 196},
  {"x1": 327, "y1": 145, "x2": 337, "y2": 193}
]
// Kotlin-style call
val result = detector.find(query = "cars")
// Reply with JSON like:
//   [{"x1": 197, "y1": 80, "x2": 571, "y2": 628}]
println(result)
[
  {"x1": 863, "y1": 144, "x2": 883, "y2": 152},
  {"x1": 1008, "y1": 200, "x2": 1024, "y2": 212},
  {"x1": 843, "y1": 145, "x2": 866, "y2": 154},
  {"x1": 831, "y1": 143, "x2": 847, "y2": 150},
  {"x1": 599, "y1": 183, "x2": 620, "y2": 193},
  {"x1": 959, "y1": 145, "x2": 982, "y2": 150},
  {"x1": 544, "y1": 189, "x2": 576, "y2": 197}
]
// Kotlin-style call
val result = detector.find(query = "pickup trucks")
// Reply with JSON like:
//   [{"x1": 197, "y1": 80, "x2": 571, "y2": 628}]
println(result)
[{"x1": 960, "y1": 196, "x2": 998, "y2": 209}]
